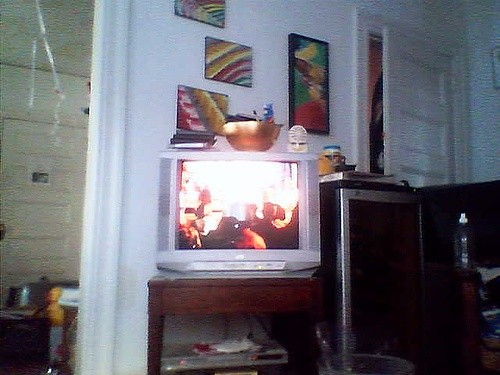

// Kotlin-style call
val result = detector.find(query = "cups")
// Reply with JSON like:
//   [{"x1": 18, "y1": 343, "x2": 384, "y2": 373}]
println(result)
[{"x1": 323, "y1": 144, "x2": 345, "y2": 167}]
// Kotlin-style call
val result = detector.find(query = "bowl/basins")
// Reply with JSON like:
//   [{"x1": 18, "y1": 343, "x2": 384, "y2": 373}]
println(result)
[{"x1": 223, "y1": 120, "x2": 284, "y2": 152}]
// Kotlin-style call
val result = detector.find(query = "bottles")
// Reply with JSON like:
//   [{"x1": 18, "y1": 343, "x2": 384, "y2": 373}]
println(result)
[{"x1": 453, "y1": 213, "x2": 473, "y2": 271}]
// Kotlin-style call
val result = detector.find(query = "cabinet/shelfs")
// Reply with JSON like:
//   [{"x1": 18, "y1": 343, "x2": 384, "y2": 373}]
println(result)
[{"x1": 320, "y1": 180, "x2": 423, "y2": 373}]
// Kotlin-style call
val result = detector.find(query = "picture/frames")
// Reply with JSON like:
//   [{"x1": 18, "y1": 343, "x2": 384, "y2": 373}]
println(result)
[
  {"x1": 177, "y1": 84, "x2": 229, "y2": 132},
  {"x1": 205, "y1": 37, "x2": 253, "y2": 89},
  {"x1": 287, "y1": 32, "x2": 330, "y2": 134},
  {"x1": 175, "y1": 0, "x2": 225, "y2": 28}
]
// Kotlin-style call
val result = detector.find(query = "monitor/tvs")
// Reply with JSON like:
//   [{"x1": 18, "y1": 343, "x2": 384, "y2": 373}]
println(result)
[{"x1": 156, "y1": 150, "x2": 320, "y2": 282}]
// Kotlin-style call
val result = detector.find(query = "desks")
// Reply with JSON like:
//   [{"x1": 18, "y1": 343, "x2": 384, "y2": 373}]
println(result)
[{"x1": 148, "y1": 274, "x2": 323, "y2": 375}]
